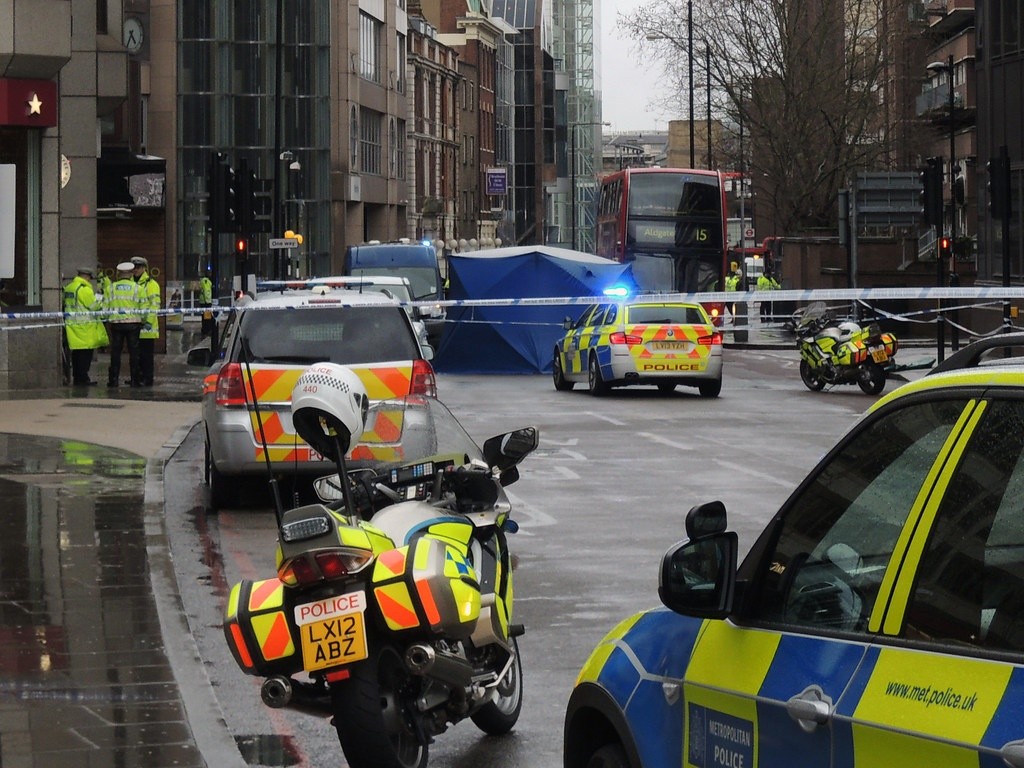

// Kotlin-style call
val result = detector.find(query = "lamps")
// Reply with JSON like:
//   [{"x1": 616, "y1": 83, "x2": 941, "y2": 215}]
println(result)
[
  {"x1": 468, "y1": 238, "x2": 477, "y2": 247},
  {"x1": 494, "y1": 238, "x2": 502, "y2": 246},
  {"x1": 458, "y1": 239, "x2": 467, "y2": 248},
  {"x1": 436, "y1": 240, "x2": 444, "y2": 249},
  {"x1": 486, "y1": 238, "x2": 492, "y2": 246},
  {"x1": 479, "y1": 238, "x2": 487, "y2": 246},
  {"x1": 449, "y1": 239, "x2": 457, "y2": 249}
]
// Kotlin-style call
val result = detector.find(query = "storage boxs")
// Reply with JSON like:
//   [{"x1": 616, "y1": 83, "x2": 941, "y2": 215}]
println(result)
[
  {"x1": 836, "y1": 341, "x2": 867, "y2": 365},
  {"x1": 367, "y1": 536, "x2": 483, "y2": 646},
  {"x1": 224, "y1": 576, "x2": 307, "y2": 678},
  {"x1": 881, "y1": 333, "x2": 899, "y2": 358}
]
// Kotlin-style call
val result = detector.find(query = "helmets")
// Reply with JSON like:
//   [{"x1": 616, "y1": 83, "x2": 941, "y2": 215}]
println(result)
[
  {"x1": 290, "y1": 361, "x2": 371, "y2": 462},
  {"x1": 838, "y1": 323, "x2": 861, "y2": 342}
]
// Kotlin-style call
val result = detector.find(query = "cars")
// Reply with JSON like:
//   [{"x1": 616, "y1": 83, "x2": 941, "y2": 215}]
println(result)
[
  {"x1": 301, "y1": 275, "x2": 433, "y2": 365},
  {"x1": 550, "y1": 289, "x2": 723, "y2": 398}
]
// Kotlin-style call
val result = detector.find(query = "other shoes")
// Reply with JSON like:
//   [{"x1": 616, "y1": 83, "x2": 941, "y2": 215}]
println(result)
[
  {"x1": 124, "y1": 380, "x2": 154, "y2": 387},
  {"x1": 106, "y1": 382, "x2": 118, "y2": 387},
  {"x1": 74, "y1": 379, "x2": 97, "y2": 384}
]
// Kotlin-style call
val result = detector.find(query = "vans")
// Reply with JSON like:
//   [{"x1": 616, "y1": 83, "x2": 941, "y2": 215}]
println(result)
[{"x1": 342, "y1": 245, "x2": 447, "y2": 341}]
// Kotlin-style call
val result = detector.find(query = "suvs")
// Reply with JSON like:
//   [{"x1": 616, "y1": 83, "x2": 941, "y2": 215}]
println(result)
[
  {"x1": 188, "y1": 290, "x2": 438, "y2": 510},
  {"x1": 562, "y1": 329, "x2": 1024, "y2": 768}
]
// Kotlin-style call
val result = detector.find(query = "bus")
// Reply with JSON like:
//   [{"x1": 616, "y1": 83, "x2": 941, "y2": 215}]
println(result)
[
  {"x1": 595, "y1": 165, "x2": 727, "y2": 323},
  {"x1": 737, "y1": 236, "x2": 784, "y2": 285}
]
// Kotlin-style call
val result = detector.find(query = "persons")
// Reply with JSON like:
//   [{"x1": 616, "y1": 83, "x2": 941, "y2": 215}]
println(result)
[
  {"x1": 726, "y1": 267, "x2": 749, "y2": 325},
  {"x1": 198, "y1": 269, "x2": 214, "y2": 333},
  {"x1": 64, "y1": 256, "x2": 161, "y2": 384},
  {"x1": 169, "y1": 289, "x2": 181, "y2": 309},
  {"x1": 757, "y1": 270, "x2": 781, "y2": 323}
]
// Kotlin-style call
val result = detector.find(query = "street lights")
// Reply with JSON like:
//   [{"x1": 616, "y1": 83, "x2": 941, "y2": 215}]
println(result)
[
  {"x1": 646, "y1": 35, "x2": 711, "y2": 170},
  {"x1": 927, "y1": 55, "x2": 976, "y2": 352}
]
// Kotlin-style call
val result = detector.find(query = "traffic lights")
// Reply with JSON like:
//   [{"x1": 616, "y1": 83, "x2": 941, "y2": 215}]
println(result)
[
  {"x1": 939, "y1": 238, "x2": 952, "y2": 258},
  {"x1": 919, "y1": 158, "x2": 942, "y2": 221},
  {"x1": 205, "y1": 164, "x2": 273, "y2": 233},
  {"x1": 235, "y1": 240, "x2": 247, "y2": 261}
]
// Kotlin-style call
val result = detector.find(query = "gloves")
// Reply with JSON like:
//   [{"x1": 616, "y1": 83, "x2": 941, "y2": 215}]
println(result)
[{"x1": 145, "y1": 322, "x2": 151, "y2": 330}]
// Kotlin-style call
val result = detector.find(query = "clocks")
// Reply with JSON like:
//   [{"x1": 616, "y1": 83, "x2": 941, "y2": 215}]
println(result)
[{"x1": 122, "y1": 17, "x2": 143, "y2": 52}]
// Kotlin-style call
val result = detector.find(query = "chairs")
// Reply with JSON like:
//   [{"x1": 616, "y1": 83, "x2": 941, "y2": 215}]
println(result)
[
  {"x1": 340, "y1": 316, "x2": 382, "y2": 361},
  {"x1": 255, "y1": 325, "x2": 295, "y2": 359}
]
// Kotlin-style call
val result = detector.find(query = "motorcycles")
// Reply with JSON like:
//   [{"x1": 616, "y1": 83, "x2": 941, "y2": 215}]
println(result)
[
  {"x1": 222, "y1": 392, "x2": 540, "y2": 768},
  {"x1": 784, "y1": 302, "x2": 898, "y2": 395}
]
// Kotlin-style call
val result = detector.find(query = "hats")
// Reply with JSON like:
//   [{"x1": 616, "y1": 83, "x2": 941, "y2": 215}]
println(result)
[
  {"x1": 130, "y1": 257, "x2": 148, "y2": 265},
  {"x1": 117, "y1": 262, "x2": 135, "y2": 272},
  {"x1": 76, "y1": 267, "x2": 95, "y2": 274}
]
